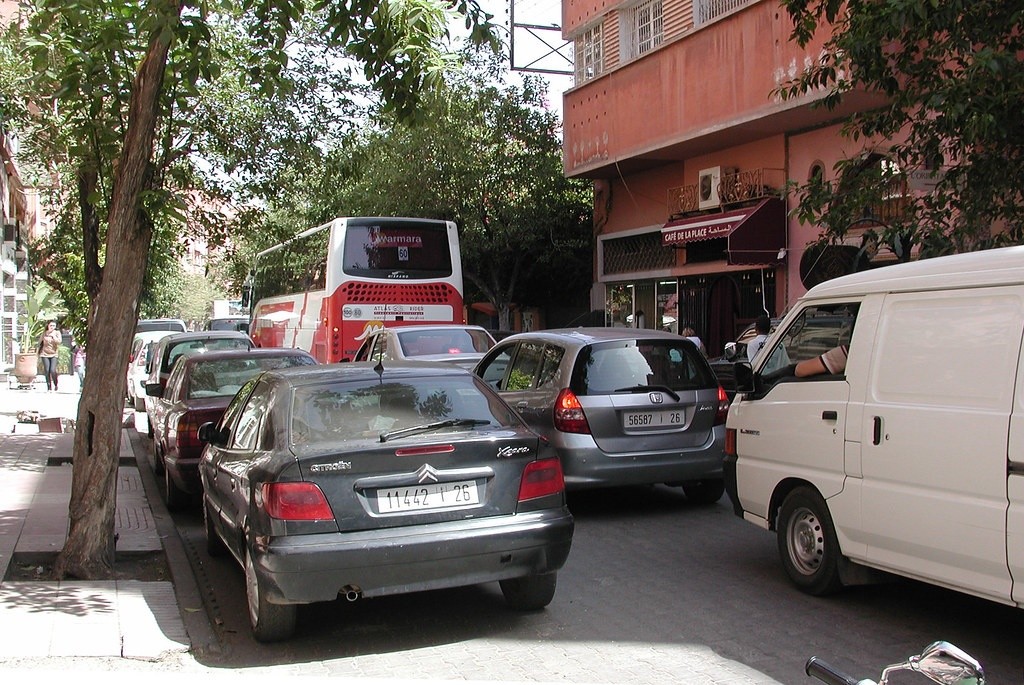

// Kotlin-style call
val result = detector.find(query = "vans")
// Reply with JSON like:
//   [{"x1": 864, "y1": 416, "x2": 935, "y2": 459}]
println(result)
[{"x1": 722, "y1": 246, "x2": 1023, "y2": 610}]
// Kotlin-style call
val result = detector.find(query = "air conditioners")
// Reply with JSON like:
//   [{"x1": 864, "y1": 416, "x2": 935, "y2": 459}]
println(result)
[{"x1": 699, "y1": 166, "x2": 735, "y2": 211}]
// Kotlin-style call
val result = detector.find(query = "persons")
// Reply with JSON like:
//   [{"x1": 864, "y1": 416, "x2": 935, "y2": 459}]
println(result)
[
  {"x1": 70, "y1": 337, "x2": 87, "y2": 392},
  {"x1": 680, "y1": 326, "x2": 709, "y2": 361},
  {"x1": 37, "y1": 319, "x2": 62, "y2": 391},
  {"x1": 746, "y1": 316, "x2": 793, "y2": 374},
  {"x1": 794, "y1": 314, "x2": 857, "y2": 377}
]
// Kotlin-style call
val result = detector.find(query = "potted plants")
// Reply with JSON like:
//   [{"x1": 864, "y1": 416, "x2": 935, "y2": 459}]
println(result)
[{"x1": 13, "y1": 281, "x2": 65, "y2": 383}]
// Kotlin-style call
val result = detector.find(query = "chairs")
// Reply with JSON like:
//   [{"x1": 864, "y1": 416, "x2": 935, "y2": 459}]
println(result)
[
  {"x1": 599, "y1": 354, "x2": 631, "y2": 379},
  {"x1": 169, "y1": 354, "x2": 184, "y2": 366},
  {"x1": 378, "y1": 387, "x2": 419, "y2": 413},
  {"x1": 192, "y1": 372, "x2": 217, "y2": 392},
  {"x1": 641, "y1": 355, "x2": 670, "y2": 374}
]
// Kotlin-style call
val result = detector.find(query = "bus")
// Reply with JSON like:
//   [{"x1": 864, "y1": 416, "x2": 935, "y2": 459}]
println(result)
[{"x1": 241, "y1": 216, "x2": 467, "y2": 365}]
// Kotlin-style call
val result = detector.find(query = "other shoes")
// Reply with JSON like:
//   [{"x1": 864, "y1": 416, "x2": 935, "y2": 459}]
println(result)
[
  {"x1": 55, "y1": 386, "x2": 57, "y2": 390},
  {"x1": 48, "y1": 390, "x2": 50, "y2": 393}
]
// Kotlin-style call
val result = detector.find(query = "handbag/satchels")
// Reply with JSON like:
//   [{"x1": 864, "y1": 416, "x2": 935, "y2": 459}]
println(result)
[{"x1": 38, "y1": 331, "x2": 44, "y2": 355}]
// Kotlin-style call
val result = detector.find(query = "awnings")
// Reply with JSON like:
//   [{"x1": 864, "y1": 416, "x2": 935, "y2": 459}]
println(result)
[{"x1": 660, "y1": 205, "x2": 756, "y2": 245}]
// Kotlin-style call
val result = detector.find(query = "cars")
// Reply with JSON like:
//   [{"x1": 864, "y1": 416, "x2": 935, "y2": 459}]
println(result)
[
  {"x1": 126, "y1": 315, "x2": 253, "y2": 439},
  {"x1": 339, "y1": 323, "x2": 510, "y2": 393},
  {"x1": 471, "y1": 326, "x2": 731, "y2": 506},
  {"x1": 197, "y1": 360, "x2": 576, "y2": 644},
  {"x1": 145, "y1": 348, "x2": 325, "y2": 513}
]
